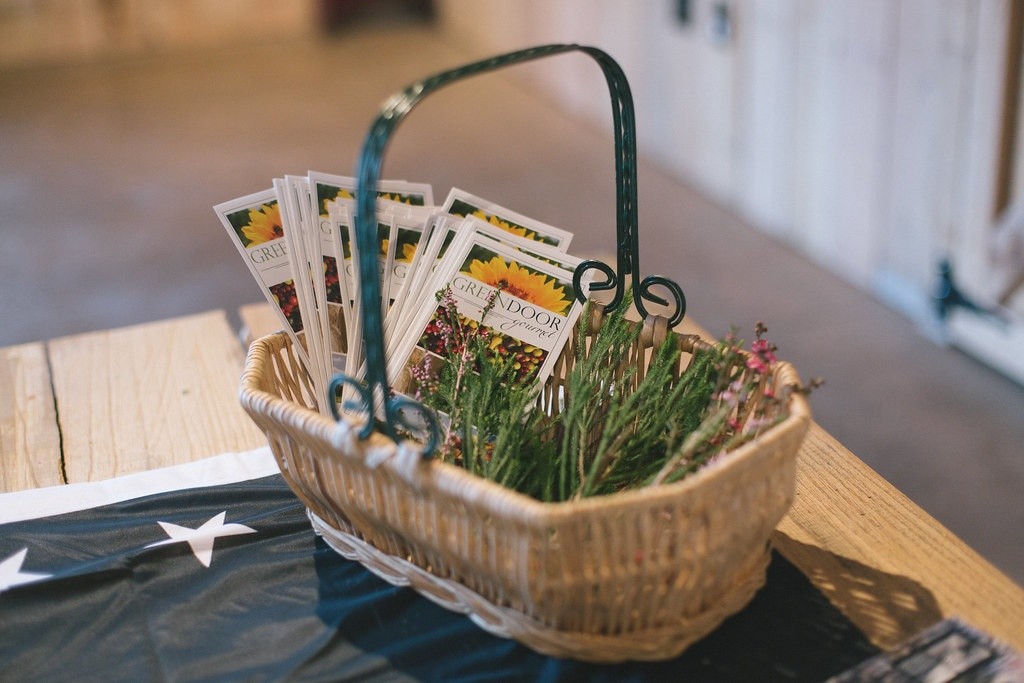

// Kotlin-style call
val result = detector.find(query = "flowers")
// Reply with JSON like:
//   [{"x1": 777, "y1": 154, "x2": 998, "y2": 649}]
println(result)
[{"x1": 404, "y1": 274, "x2": 827, "y2": 503}]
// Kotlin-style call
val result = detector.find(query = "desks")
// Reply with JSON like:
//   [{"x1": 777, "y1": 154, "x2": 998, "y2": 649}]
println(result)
[{"x1": 0, "y1": 252, "x2": 1024, "y2": 683}]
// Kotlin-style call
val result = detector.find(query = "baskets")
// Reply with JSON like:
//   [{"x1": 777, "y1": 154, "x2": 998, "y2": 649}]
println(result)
[{"x1": 235, "y1": 43, "x2": 812, "y2": 662}]
